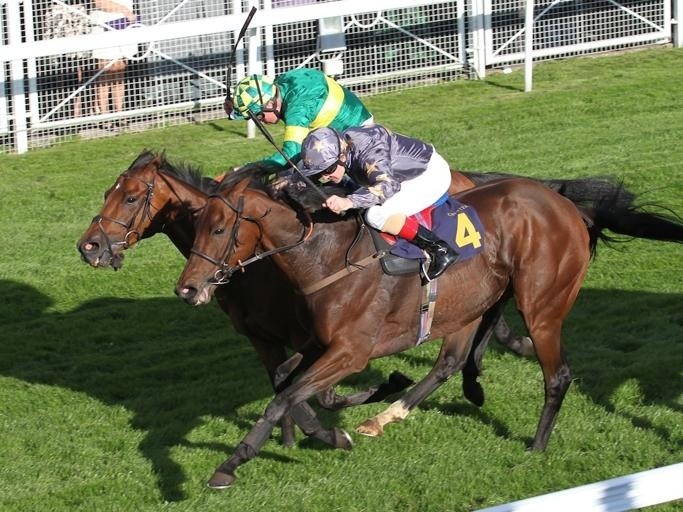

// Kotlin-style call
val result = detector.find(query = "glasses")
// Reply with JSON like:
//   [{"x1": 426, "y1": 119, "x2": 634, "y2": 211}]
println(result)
[
  {"x1": 253, "y1": 112, "x2": 265, "y2": 122},
  {"x1": 319, "y1": 162, "x2": 340, "y2": 176}
]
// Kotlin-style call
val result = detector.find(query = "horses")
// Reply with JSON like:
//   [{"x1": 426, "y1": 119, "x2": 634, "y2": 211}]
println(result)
[
  {"x1": 76, "y1": 148, "x2": 636, "y2": 446},
  {"x1": 174, "y1": 166, "x2": 683, "y2": 489}
]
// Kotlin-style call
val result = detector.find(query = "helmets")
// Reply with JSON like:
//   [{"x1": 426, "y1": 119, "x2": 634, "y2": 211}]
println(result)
[
  {"x1": 292, "y1": 126, "x2": 348, "y2": 178},
  {"x1": 229, "y1": 74, "x2": 278, "y2": 122}
]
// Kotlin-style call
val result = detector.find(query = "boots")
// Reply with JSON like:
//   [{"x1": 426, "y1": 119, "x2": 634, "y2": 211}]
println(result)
[{"x1": 410, "y1": 225, "x2": 460, "y2": 285}]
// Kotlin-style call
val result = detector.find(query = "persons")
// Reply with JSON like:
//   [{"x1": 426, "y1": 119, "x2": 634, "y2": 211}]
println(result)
[
  {"x1": 86, "y1": 0, "x2": 140, "y2": 118},
  {"x1": 263, "y1": 122, "x2": 463, "y2": 282},
  {"x1": 229, "y1": 66, "x2": 377, "y2": 182}
]
